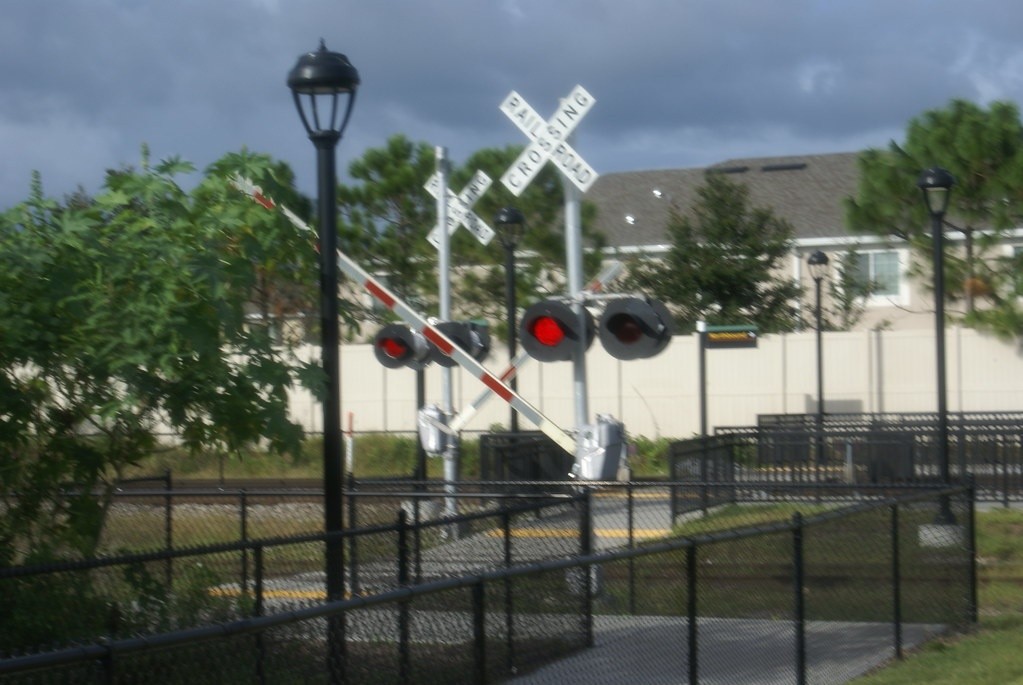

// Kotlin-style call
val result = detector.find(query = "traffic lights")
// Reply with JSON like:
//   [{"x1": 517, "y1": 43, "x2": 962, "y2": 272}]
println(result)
[
  {"x1": 373, "y1": 324, "x2": 416, "y2": 370},
  {"x1": 598, "y1": 295, "x2": 660, "y2": 361},
  {"x1": 426, "y1": 321, "x2": 472, "y2": 367},
  {"x1": 519, "y1": 299, "x2": 580, "y2": 364}
]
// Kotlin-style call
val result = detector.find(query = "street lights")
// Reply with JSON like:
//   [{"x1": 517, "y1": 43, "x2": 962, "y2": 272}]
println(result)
[
  {"x1": 806, "y1": 249, "x2": 831, "y2": 503},
  {"x1": 281, "y1": 35, "x2": 364, "y2": 685},
  {"x1": 494, "y1": 206, "x2": 529, "y2": 434},
  {"x1": 920, "y1": 166, "x2": 959, "y2": 528}
]
